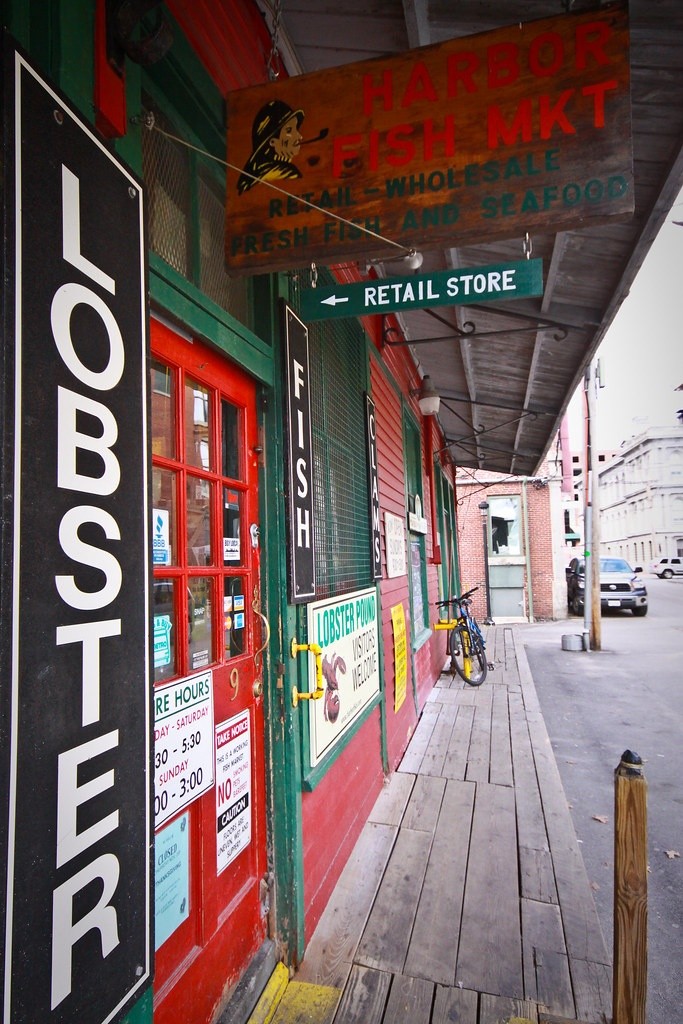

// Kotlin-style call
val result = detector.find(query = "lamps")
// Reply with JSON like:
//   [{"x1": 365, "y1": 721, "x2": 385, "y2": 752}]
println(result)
[
  {"x1": 478, "y1": 501, "x2": 489, "y2": 520},
  {"x1": 410, "y1": 374, "x2": 441, "y2": 415}
]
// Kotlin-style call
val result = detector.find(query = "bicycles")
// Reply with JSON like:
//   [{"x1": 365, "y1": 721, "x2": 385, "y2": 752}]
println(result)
[{"x1": 434, "y1": 587, "x2": 488, "y2": 686}]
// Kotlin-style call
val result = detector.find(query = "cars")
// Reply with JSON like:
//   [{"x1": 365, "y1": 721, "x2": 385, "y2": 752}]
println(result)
[
  {"x1": 153, "y1": 583, "x2": 195, "y2": 642},
  {"x1": 567, "y1": 556, "x2": 648, "y2": 616}
]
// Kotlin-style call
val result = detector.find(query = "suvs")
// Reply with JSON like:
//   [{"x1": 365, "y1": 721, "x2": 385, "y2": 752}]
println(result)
[{"x1": 650, "y1": 556, "x2": 683, "y2": 579}]
